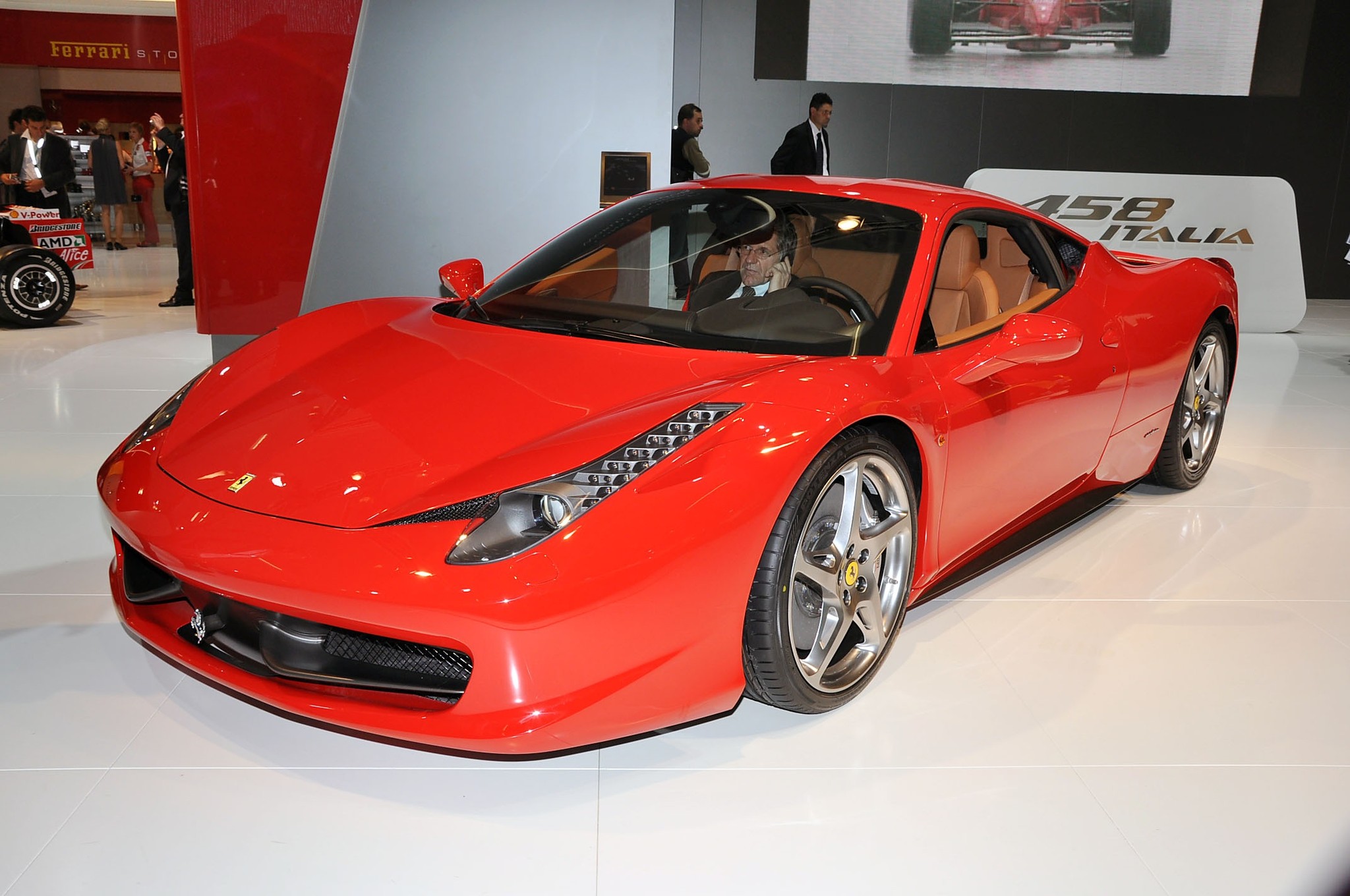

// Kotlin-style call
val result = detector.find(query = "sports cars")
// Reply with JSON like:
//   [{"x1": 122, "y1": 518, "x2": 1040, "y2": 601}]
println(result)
[{"x1": 94, "y1": 173, "x2": 1242, "y2": 764}]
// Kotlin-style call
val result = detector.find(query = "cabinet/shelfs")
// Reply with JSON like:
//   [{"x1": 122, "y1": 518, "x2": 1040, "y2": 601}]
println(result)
[{"x1": 59, "y1": 135, "x2": 115, "y2": 234}]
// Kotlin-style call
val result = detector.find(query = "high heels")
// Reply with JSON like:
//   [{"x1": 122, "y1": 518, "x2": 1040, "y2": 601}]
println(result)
[
  {"x1": 107, "y1": 242, "x2": 113, "y2": 250},
  {"x1": 114, "y1": 242, "x2": 127, "y2": 250}
]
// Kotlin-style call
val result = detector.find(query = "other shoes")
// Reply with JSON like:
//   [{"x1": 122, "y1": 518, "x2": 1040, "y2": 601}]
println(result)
[
  {"x1": 137, "y1": 240, "x2": 157, "y2": 247},
  {"x1": 674, "y1": 287, "x2": 689, "y2": 300}
]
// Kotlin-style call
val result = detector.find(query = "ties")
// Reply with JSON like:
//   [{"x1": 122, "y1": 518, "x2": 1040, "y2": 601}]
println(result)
[
  {"x1": 816, "y1": 132, "x2": 823, "y2": 175},
  {"x1": 740, "y1": 285, "x2": 756, "y2": 297}
]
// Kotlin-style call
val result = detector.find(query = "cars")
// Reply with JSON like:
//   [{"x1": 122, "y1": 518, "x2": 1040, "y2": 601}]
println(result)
[{"x1": 0, "y1": 214, "x2": 77, "y2": 330}]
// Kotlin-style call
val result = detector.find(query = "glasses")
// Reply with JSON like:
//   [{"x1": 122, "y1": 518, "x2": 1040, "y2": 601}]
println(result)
[{"x1": 735, "y1": 242, "x2": 783, "y2": 261}]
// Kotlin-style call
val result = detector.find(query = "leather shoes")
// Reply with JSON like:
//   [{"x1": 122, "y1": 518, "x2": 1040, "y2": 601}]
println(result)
[{"x1": 158, "y1": 295, "x2": 192, "y2": 307}]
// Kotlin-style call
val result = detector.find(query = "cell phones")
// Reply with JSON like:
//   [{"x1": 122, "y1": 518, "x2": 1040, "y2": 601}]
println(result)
[{"x1": 780, "y1": 252, "x2": 794, "y2": 267}]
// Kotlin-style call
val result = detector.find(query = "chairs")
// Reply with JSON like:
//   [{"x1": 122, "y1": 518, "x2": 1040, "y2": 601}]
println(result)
[
  {"x1": 737, "y1": 217, "x2": 827, "y2": 305},
  {"x1": 981, "y1": 223, "x2": 1048, "y2": 313},
  {"x1": 874, "y1": 224, "x2": 999, "y2": 337}
]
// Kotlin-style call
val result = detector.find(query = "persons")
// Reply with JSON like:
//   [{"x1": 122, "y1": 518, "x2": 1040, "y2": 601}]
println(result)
[
  {"x1": 687, "y1": 212, "x2": 825, "y2": 311},
  {"x1": 0, "y1": 109, "x2": 196, "y2": 308},
  {"x1": 672, "y1": 103, "x2": 712, "y2": 299},
  {"x1": 769, "y1": 91, "x2": 832, "y2": 225}
]
